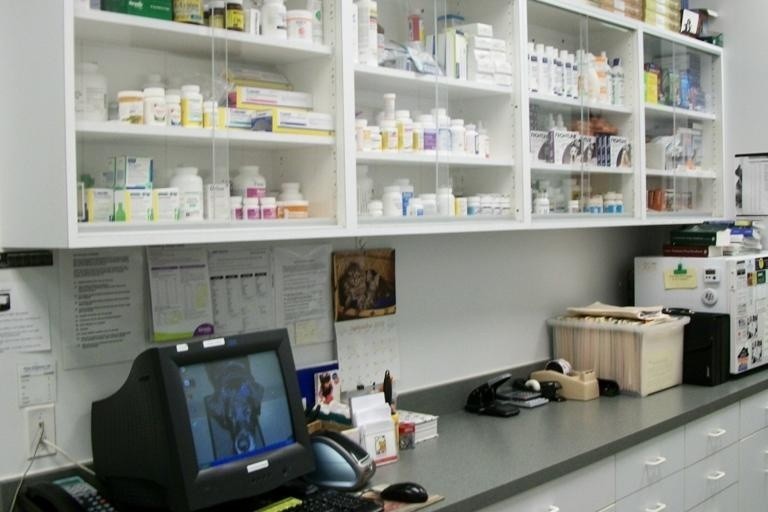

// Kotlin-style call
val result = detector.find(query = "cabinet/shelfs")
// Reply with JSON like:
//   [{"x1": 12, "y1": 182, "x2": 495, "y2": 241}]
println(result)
[
  {"x1": 0, "y1": 356, "x2": 768, "y2": 510},
  {"x1": 0, "y1": 1, "x2": 725, "y2": 250}
]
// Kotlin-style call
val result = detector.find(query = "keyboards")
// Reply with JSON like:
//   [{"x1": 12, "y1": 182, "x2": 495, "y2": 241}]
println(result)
[{"x1": 278, "y1": 488, "x2": 383, "y2": 512}]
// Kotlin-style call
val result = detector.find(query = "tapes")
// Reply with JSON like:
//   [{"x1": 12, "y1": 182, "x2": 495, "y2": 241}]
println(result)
[
  {"x1": 544, "y1": 358, "x2": 572, "y2": 376},
  {"x1": 524, "y1": 379, "x2": 540, "y2": 392}
]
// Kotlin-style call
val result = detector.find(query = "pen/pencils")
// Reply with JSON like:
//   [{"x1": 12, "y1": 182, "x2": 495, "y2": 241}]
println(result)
[{"x1": 384, "y1": 370, "x2": 391, "y2": 405}]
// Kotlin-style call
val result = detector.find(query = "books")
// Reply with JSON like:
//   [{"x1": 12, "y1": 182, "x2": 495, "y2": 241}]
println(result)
[{"x1": 566, "y1": 301, "x2": 664, "y2": 320}]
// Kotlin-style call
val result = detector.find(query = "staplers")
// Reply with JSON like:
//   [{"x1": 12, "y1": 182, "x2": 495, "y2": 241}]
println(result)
[{"x1": 540, "y1": 381, "x2": 566, "y2": 402}]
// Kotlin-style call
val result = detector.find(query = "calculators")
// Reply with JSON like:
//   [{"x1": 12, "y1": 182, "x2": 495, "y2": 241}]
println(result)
[{"x1": 497, "y1": 386, "x2": 542, "y2": 401}]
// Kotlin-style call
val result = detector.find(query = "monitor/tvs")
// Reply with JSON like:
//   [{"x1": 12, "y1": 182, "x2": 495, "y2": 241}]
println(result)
[{"x1": 90, "y1": 326, "x2": 316, "y2": 512}]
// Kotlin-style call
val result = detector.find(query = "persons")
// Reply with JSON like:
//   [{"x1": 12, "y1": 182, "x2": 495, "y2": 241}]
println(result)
[{"x1": 318, "y1": 372, "x2": 334, "y2": 405}]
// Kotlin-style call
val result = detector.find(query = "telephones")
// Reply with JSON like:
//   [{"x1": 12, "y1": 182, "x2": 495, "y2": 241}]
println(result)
[{"x1": 16, "y1": 475, "x2": 119, "y2": 512}]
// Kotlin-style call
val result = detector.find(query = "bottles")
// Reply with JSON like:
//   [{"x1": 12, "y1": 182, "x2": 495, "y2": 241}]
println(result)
[
  {"x1": 171, "y1": 167, "x2": 203, "y2": 223},
  {"x1": 75, "y1": 62, "x2": 108, "y2": 122},
  {"x1": 530, "y1": 176, "x2": 624, "y2": 217},
  {"x1": 355, "y1": 90, "x2": 513, "y2": 220},
  {"x1": 229, "y1": 165, "x2": 308, "y2": 226},
  {"x1": 116, "y1": 83, "x2": 205, "y2": 131},
  {"x1": 409, "y1": 7, "x2": 424, "y2": 43},
  {"x1": 528, "y1": 40, "x2": 626, "y2": 110},
  {"x1": 173, "y1": 0, "x2": 314, "y2": 43}
]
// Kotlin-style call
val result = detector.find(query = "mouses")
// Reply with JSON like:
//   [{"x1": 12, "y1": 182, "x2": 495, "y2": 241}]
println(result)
[{"x1": 380, "y1": 482, "x2": 428, "y2": 503}]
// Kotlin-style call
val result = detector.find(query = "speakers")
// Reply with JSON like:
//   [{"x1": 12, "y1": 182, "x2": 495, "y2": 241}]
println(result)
[{"x1": 310, "y1": 428, "x2": 376, "y2": 490}]
